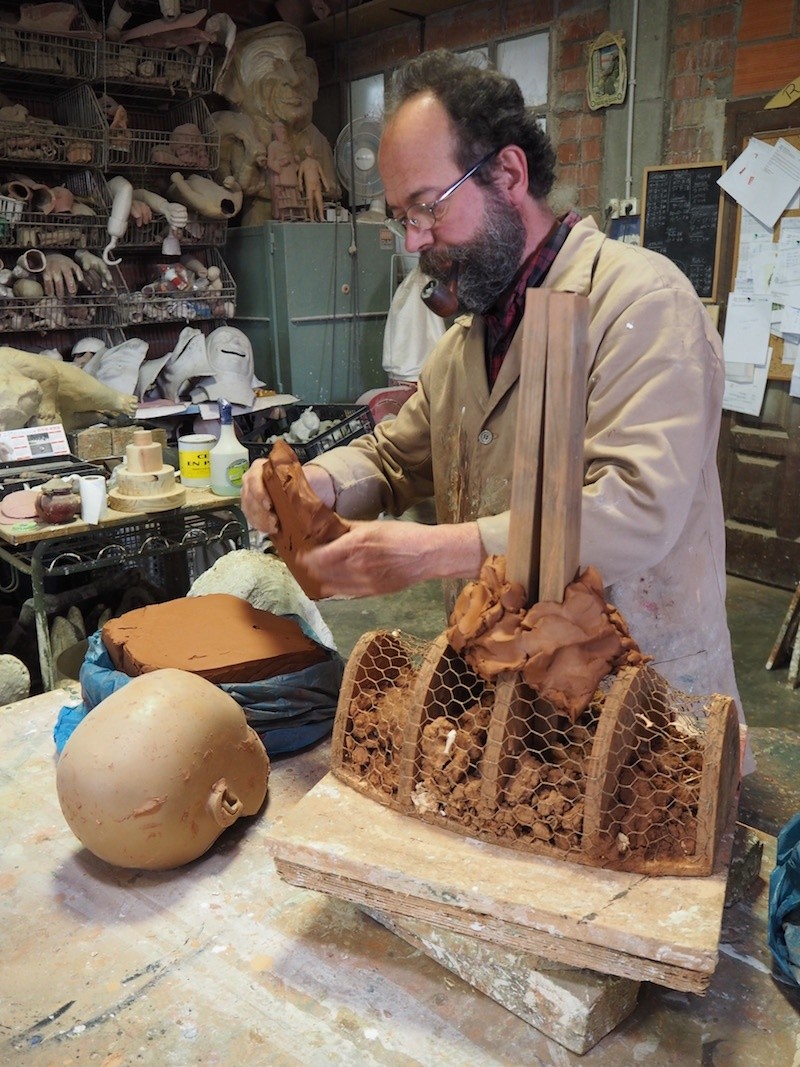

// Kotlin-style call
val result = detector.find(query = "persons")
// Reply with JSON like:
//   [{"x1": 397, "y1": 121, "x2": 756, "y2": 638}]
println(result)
[
  {"x1": 241, "y1": 50, "x2": 759, "y2": 779},
  {"x1": 1, "y1": 0, "x2": 340, "y2": 874}
]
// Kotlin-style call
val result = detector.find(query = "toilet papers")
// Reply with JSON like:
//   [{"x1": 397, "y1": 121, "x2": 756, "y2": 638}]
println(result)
[{"x1": 78, "y1": 476, "x2": 109, "y2": 525}]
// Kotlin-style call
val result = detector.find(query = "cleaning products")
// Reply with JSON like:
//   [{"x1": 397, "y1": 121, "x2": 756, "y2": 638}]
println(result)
[{"x1": 206, "y1": 396, "x2": 252, "y2": 497}]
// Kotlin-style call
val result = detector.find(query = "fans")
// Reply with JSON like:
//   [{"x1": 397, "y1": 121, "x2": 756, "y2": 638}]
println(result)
[{"x1": 334, "y1": 116, "x2": 388, "y2": 226}]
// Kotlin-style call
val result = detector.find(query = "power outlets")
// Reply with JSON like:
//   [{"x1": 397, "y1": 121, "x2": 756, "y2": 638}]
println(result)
[{"x1": 609, "y1": 198, "x2": 641, "y2": 219}]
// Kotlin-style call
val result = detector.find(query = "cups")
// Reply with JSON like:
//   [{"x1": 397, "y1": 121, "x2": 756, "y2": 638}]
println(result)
[{"x1": 178, "y1": 433, "x2": 218, "y2": 488}]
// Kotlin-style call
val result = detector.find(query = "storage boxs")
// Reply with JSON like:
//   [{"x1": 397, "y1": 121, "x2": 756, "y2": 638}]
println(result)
[{"x1": 239, "y1": 403, "x2": 375, "y2": 469}]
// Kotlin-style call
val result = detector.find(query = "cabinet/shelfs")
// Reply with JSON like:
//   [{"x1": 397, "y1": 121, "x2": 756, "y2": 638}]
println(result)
[{"x1": 1, "y1": 2, "x2": 238, "y2": 350}]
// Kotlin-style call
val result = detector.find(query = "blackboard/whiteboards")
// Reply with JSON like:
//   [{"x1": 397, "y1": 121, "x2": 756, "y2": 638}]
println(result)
[{"x1": 639, "y1": 159, "x2": 728, "y2": 303}]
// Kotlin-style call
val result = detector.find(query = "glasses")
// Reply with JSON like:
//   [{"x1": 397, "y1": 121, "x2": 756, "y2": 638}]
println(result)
[{"x1": 384, "y1": 142, "x2": 508, "y2": 239}]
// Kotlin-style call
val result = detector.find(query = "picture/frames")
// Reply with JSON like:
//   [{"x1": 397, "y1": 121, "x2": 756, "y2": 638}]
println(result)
[{"x1": 584, "y1": 30, "x2": 628, "y2": 111}]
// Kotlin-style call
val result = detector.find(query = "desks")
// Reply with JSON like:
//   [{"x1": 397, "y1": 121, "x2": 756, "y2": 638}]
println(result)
[{"x1": 0, "y1": 478, "x2": 251, "y2": 692}]
